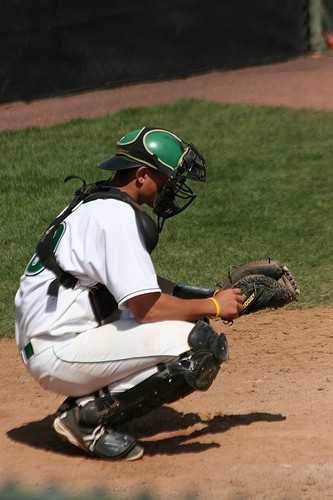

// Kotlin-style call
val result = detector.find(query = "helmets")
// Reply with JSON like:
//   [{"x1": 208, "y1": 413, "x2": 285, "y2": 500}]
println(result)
[{"x1": 96, "y1": 127, "x2": 206, "y2": 183}]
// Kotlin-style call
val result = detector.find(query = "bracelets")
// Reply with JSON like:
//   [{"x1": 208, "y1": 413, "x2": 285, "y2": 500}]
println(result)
[{"x1": 206, "y1": 297, "x2": 219, "y2": 319}]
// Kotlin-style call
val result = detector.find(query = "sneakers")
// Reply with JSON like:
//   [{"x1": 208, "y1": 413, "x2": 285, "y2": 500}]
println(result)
[{"x1": 53, "y1": 396, "x2": 145, "y2": 462}]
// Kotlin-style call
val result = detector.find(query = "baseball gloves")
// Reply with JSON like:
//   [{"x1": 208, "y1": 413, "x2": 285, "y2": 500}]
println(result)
[{"x1": 213, "y1": 256, "x2": 300, "y2": 327}]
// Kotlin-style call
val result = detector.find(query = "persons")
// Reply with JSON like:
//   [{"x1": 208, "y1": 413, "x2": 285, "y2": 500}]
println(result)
[{"x1": 14, "y1": 127, "x2": 246, "y2": 460}]
